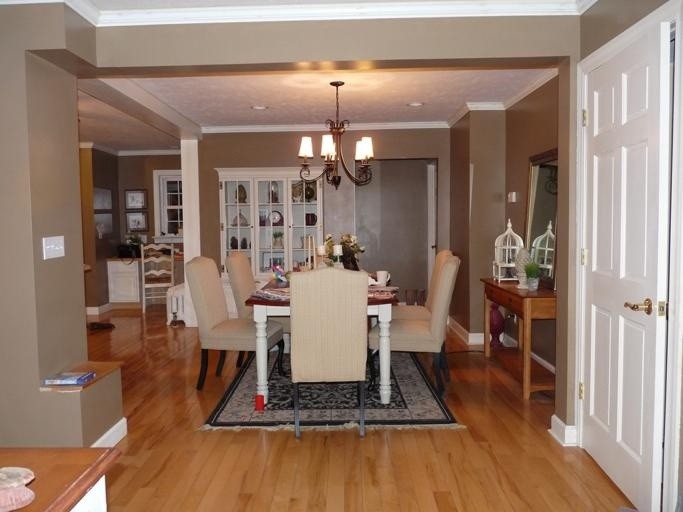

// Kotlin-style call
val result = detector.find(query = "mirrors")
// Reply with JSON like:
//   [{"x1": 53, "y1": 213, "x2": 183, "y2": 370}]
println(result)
[{"x1": 522, "y1": 148, "x2": 559, "y2": 290}]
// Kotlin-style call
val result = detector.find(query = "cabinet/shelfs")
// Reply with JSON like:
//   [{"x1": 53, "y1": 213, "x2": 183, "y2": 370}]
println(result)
[
  {"x1": 214, "y1": 166, "x2": 324, "y2": 320},
  {"x1": 107, "y1": 251, "x2": 184, "y2": 317},
  {"x1": 159, "y1": 175, "x2": 183, "y2": 236}
]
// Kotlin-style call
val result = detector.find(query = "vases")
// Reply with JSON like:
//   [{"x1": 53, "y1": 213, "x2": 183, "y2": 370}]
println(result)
[{"x1": 344, "y1": 256, "x2": 359, "y2": 271}]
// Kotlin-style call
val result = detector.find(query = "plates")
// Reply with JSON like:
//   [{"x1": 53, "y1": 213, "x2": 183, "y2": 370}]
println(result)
[
  {"x1": 1, "y1": 466, "x2": 37, "y2": 487},
  {"x1": 1, "y1": 487, "x2": 37, "y2": 512}
]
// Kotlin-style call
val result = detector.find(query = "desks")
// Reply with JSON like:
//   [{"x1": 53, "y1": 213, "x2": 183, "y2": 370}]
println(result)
[{"x1": 480, "y1": 277, "x2": 557, "y2": 400}]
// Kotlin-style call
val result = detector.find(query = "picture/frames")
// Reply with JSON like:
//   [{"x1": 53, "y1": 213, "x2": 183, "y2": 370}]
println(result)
[
  {"x1": 123, "y1": 233, "x2": 148, "y2": 245},
  {"x1": 124, "y1": 210, "x2": 149, "y2": 232},
  {"x1": 262, "y1": 252, "x2": 284, "y2": 271},
  {"x1": 124, "y1": 188, "x2": 147, "y2": 210}
]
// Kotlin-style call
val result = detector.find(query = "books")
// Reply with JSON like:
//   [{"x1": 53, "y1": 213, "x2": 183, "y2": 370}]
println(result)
[{"x1": 44, "y1": 371, "x2": 96, "y2": 385}]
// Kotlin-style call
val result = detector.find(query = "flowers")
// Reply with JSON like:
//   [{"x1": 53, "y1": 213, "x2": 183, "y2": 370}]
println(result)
[{"x1": 341, "y1": 234, "x2": 364, "y2": 262}]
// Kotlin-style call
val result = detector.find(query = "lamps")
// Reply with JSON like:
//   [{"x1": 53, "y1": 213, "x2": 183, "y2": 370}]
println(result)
[{"x1": 297, "y1": 80, "x2": 374, "y2": 189}]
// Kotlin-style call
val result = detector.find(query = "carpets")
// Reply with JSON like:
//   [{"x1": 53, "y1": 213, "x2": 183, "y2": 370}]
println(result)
[{"x1": 195, "y1": 352, "x2": 467, "y2": 433}]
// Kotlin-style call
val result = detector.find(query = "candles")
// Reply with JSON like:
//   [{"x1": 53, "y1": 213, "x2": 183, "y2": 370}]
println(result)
[
  {"x1": 317, "y1": 245, "x2": 326, "y2": 261},
  {"x1": 331, "y1": 244, "x2": 343, "y2": 261}
]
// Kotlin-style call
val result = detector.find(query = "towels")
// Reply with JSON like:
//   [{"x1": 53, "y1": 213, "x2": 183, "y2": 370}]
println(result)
[
  {"x1": 367, "y1": 286, "x2": 399, "y2": 301},
  {"x1": 251, "y1": 288, "x2": 292, "y2": 303}
]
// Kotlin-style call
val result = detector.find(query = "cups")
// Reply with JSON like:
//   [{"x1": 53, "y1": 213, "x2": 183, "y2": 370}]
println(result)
[
  {"x1": 0, "y1": 470, "x2": 28, "y2": 507},
  {"x1": 377, "y1": 271, "x2": 392, "y2": 286}
]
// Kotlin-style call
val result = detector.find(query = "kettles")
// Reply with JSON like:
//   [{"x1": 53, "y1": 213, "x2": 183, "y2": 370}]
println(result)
[{"x1": 306, "y1": 214, "x2": 318, "y2": 226}]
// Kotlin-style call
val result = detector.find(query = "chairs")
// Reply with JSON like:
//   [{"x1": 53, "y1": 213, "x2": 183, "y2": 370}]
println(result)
[
  {"x1": 370, "y1": 249, "x2": 461, "y2": 398},
  {"x1": 224, "y1": 253, "x2": 289, "y2": 370},
  {"x1": 290, "y1": 269, "x2": 369, "y2": 438},
  {"x1": 185, "y1": 256, "x2": 286, "y2": 390}
]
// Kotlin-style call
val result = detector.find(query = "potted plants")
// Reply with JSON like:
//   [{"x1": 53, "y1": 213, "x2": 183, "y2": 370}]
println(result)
[
  {"x1": 524, "y1": 262, "x2": 541, "y2": 291},
  {"x1": 272, "y1": 232, "x2": 284, "y2": 249}
]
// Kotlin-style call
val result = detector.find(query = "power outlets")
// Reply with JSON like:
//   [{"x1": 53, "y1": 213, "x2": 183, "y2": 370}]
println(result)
[{"x1": 513, "y1": 313, "x2": 518, "y2": 324}]
